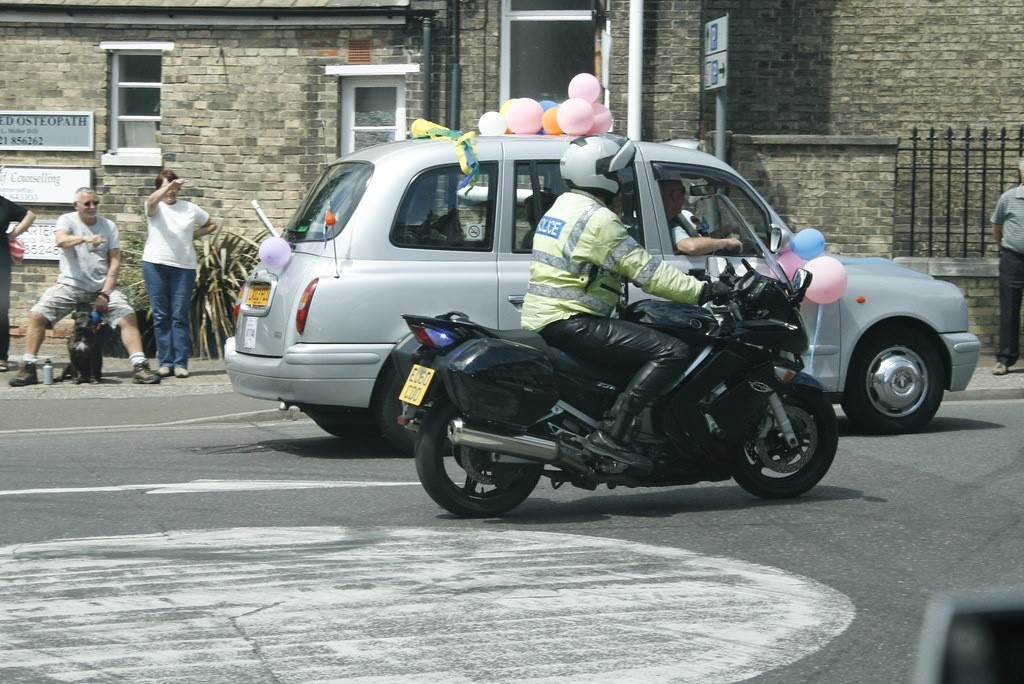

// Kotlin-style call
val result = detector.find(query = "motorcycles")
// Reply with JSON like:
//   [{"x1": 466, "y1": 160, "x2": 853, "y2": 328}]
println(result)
[{"x1": 398, "y1": 194, "x2": 840, "y2": 519}]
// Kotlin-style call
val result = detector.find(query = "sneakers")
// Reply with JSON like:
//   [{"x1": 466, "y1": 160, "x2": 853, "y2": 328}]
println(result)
[
  {"x1": 9, "y1": 362, "x2": 37, "y2": 386},
  {"x1": 133, "y1": 361, "x2": 159, "y2": 383}
]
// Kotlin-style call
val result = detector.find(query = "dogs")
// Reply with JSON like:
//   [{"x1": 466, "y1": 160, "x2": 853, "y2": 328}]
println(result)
[{"x1": 66, "y1": 309, "x2": 104, "y2": 385}]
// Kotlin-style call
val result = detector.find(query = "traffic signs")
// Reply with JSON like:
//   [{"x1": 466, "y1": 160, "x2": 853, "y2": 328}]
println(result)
[{"x1": 703, "y1": 14, "x2": 730, "y2": 93}]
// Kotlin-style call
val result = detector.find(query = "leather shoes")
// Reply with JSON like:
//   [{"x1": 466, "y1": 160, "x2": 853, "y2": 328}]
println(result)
[{"x1": 585, "y1": 430, "x2": 654, "y2": 469}]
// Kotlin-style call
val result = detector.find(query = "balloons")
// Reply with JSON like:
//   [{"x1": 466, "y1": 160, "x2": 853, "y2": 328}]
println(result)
[
  {"x1": 259, "y1": 237, "x2": 292, "y2": 269},
  {"x1": 770, "y1": 250, "x2": 804, "y2": 281},
  {"x1": 803, "y1": 256, "x2": 848, "y2": 305},
  {"x1": 791, "y1": 228, "x2": 825, "y2": 260},
  {"x1": 325, "y1": 210, "x2": 336, "y2": 226},
  {"x1": 478, "y1": 72, "x2": 612, "y2": 136}
]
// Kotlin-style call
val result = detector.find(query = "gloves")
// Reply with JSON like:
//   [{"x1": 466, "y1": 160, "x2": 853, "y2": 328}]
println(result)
[{"x1": 698, "y1": 282, "x2": 731, "y2": 307}]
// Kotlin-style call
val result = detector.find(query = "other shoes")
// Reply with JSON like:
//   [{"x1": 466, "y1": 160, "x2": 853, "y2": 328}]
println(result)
[
  {"x1": 156, "y1": 366, "x2": 173, "y2": 377},
  {"x1": 0, "y1": 360, "x2": 8, "y2": 370},
  {"x1": 174, "y1": 367, "x2": 189, "y2": 377},
  {"x1": 992, "y1": 361, "x2": 1008, "y2": 374}
]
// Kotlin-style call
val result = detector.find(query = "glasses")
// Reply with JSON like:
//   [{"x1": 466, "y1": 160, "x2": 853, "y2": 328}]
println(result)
[
  {"x1": 670, "y1": 187, "x2": 685, "y2": 196},
  {"x1": 77, "y1": 201, "x2": 99, "y2": 206}
]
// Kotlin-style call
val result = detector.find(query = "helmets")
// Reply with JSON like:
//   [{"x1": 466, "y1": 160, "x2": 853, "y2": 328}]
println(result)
[{"x1": 559, "y1": 135, "x2": 635, "y2": 198}]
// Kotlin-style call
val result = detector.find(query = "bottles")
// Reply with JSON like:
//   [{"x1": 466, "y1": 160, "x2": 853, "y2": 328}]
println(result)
[{"x1": 42, "y1": 358, "x2": 54, "y2": 385}]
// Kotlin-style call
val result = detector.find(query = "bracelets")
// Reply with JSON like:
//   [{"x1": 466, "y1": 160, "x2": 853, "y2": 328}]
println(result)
[{"x1": 98, "y1": 292, "x2": 109, "y2": 303}]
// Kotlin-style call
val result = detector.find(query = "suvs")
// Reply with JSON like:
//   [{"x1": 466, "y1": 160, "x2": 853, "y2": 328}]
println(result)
[{"x1": 224, "y1": 134, "x2": 981, "y2": 459}]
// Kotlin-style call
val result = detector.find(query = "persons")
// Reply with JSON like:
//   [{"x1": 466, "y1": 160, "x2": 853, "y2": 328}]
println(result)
[
  {"x1": 142, "y1": 170, "x2": 218, "y2": 378},
  {"x1": 990, "y1": 156, "x2": 1024, "y2": 375},
  {"x1": 522, "y1": 133, "x2": 732, "y2": 471},
  {"x1": 7, "y1": 186, "x2": 162, "y2": 387},
  {"x1": 657, "y1": 179, "x2": 742, "y2": 255},
  {"x1": 0, "y1": 196, "x2": 36, "y2": 373}
]
